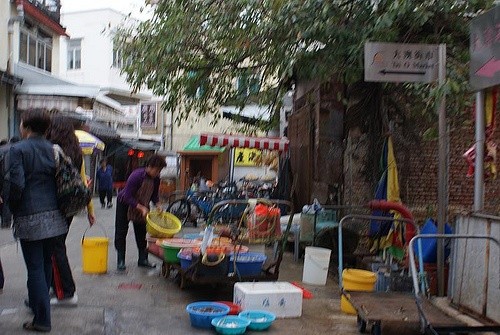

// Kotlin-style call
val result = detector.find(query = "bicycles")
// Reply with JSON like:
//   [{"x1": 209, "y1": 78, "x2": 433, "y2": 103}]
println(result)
[{"x1": 166, "y1": 175, "x2": 277, "y2": 228}]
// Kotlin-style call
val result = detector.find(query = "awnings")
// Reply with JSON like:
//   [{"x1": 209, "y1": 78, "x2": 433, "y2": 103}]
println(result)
[
  {"x1": 88, "y1": 124, "x2": 118, "y2": 137},
  {"x1": 198, "y1": 132, "x2": 291, "y2": 198}
]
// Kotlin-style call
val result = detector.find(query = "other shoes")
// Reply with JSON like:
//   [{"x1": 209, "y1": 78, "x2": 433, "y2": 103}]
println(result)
[
  {"x1": 50, "y1": 293, "x2": 79, "y2": 306},
  {"x1": 23, "y1": 320, "x2": 51, "y2": 332},
  {"x1": 49, "y1": 286, "x2": 53, "y2": 293},
  {"x1": 24, "y1": 299, "x2": 28, "y2": 306},
  {"x1": 101, "y1": 203, "x2": 105, "y2": 207},
  {"x1": 107, "y1": 202, "x2": 113, "y2": 207}
]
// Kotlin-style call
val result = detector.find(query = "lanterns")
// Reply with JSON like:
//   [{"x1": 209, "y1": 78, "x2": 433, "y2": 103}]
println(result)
[
  {"x1": 127, "y1": 149, "x2": 135, "y2": 156},
  {"x1": 137, "y1": 151, "x2": 145, "y2": 158}
]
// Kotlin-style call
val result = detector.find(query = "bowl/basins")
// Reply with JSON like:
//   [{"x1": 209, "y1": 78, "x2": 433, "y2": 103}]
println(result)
[
  {"x1": 145, "y1": 234, "x2": 267, "y2": 278},
  {"x1": 186, "y1": 301, "x2": 230, "y2": 329},
  {"x1": 211, "y1": 299, "x2": 275, "y2": 335}
]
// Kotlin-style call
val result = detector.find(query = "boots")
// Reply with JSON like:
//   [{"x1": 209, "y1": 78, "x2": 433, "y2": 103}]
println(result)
[
  {"x1": 117, "y1": 247, "x2": 126, "y2": 270},
  {"x1": 138, "y1": 249, "x2": 156, "y2": 268}
]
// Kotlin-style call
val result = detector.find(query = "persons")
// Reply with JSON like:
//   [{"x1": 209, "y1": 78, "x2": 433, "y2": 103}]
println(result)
[
  {"x1": 185, "y1": 167, "x2": 213, "y2": 190},
  {"x1": 6, "y1": 106, "x2": 90, "y2": 335},
  {"x1": 112, "y1": 152, "x2": 168, "y2": 272},
  {"x1": 95, "y1": 157, "x2": 114, "y2": 209},
  {"x1": 49, "y1": 115, "x2": 97, "y2": 308},
  {"x1": 0, "y1": 134, "x2": 21, "y2": 229},
  {"x1": 0, "y1": 259, "x2": 6, "y2": 296}
]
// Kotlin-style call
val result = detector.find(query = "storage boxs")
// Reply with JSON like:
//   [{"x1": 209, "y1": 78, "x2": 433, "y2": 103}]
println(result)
[
  {"x1": 363, "y1": 257, "x2": 399, "y2": 293},
  {"x1": 419, "y1": 261, "x2": 449, "y2": 294},
  {"x1": 233, "y1": 280, "x2": 304, "y2": 318}
]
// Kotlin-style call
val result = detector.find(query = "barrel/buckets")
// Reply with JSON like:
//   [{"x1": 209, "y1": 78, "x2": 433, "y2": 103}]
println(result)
[
  {"x1": 302, "y1": 246, "x2": 332, "y2": 287},
  {"x1": 339, "y1": 267, "x2": 379, "y2": 314},
  {"x1": 299, "y1": 205, "x2": 317, "y2": 242},
  {"x1": 81, "y1": 221, "x2": 109, "y2": 275},
  {"x1": 411, "y1": 219, "x2": 455, "y2": 263}
]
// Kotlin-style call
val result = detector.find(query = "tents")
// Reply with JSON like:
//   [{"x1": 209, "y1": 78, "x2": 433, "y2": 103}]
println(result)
[{"x1": 74, "y1": 129, "x2": 106, "y2": 197}]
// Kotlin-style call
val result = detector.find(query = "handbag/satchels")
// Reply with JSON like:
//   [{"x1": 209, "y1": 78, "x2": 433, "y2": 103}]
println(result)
[{"x1": 53, "y1": 143, "x2": 92, "y2": 218}]
